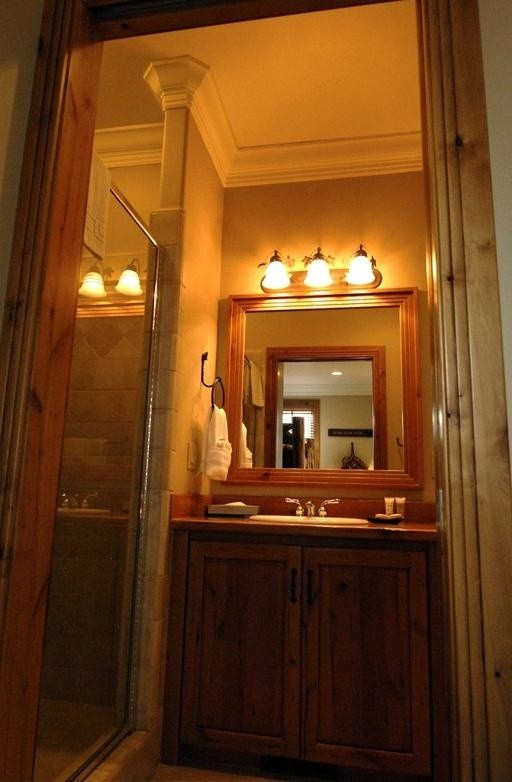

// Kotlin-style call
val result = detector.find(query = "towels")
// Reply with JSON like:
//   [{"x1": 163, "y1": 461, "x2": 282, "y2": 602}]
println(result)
[
  {"x1": 198, "y1": 402, "x2": 235, "y2": 485},
  {"x1": 81, "y1": 149, "x2": 114, "y2": 262},
  {"x1": 240, "y1": 422, "x2": 255, "y2": 469},
  {"x1": 244, "y1": 359, "x2": 266, "y2": 408}
]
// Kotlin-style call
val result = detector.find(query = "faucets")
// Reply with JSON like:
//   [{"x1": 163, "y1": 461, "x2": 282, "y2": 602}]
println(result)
[
  {"x1": 305, "y1": 502, "x2": 316, "y2": 516},
  {"x1": 80, "y1": 492, "x2": 98, "y2": 507},
  {"x1": 58, "y1": 489, "x2": 79, "y2": 507}
]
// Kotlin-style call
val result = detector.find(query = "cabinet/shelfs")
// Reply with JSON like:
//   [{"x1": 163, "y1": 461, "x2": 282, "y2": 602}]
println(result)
[{"x1": 163, "y1": 514, "x2": 462, "y2": 782}]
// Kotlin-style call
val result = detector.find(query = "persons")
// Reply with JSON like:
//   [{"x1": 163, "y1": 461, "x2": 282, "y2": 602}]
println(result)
[{"x1": 284, "y1": 425, "x2": 298, "y2": 467}]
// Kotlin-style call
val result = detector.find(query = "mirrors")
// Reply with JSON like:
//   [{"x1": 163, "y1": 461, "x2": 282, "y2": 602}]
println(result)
[
  {"x1": 222, "y1": 286, "x2": 426, "y2": 493},
  {"x1": 29, "y1": 171, "x2": 163, "y2": 781}
]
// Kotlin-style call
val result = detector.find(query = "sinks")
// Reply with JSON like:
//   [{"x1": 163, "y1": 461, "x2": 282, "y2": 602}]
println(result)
[
  {"x1": 249, "y1": 514, "x2": 369, "y2": 527},
  {"x1": 57, "y1": 508, "x2": 113, "y2": 516}
]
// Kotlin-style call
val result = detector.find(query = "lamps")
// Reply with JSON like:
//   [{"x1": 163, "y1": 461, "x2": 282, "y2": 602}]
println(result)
[
  {"x1": 77, "y1": 254, "x2": 154, "y2": 302},
  {"x1": 254, "y1": 242, "x2": 384, "y2": 295}
]
